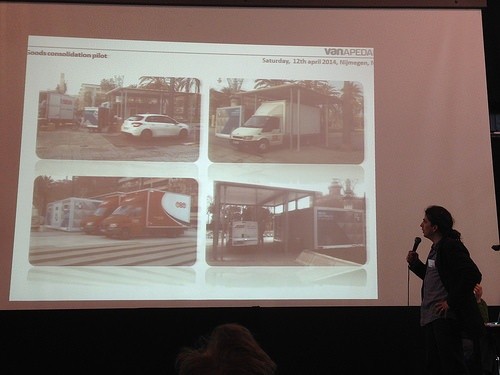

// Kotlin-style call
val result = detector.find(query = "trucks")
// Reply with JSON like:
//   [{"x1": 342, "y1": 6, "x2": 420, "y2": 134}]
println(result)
[
  {"x1": 229, "y1": 99, "x2": 324, "y2": 156},
  {"x1": 80, "y1": 188, "x2": 192, "y2": 241},
  {"x1": 223, "y1": 219, "x2": 261, "y2": 253},
  {"x1": 38, "y1": 89, "x2": 81, "y2": 128}
]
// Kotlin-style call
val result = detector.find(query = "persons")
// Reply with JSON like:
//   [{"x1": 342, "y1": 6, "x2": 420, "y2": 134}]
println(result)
[
  {"x1": 406, "y1": 204, "x2": 484, "y2": 375},
  {"x1": 474, "y1": 284, "x2": 490, "y2": 325},
  {"x1": 176, "y1": 323, "x2": 278, "y2": 375}
]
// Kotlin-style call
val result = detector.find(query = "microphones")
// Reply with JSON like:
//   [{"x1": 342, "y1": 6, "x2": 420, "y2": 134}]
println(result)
[{"x1": 412, "y1": 237, "x2": 421, "y2": 251}]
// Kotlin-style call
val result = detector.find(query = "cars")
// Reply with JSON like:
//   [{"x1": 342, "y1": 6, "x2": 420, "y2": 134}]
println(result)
[{"x1": 119, "y1": 112, "x2": 192, "y2": 142}]
